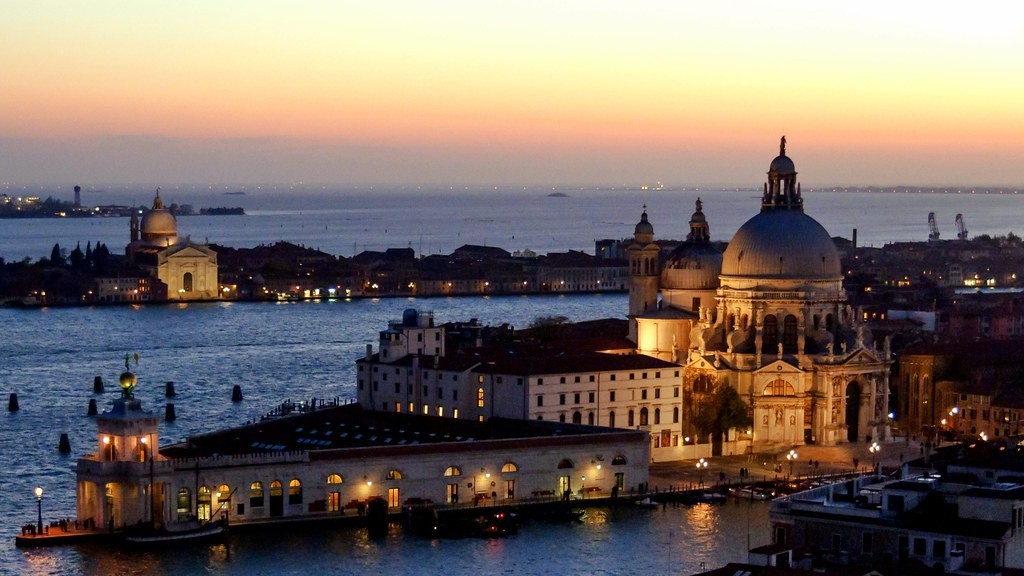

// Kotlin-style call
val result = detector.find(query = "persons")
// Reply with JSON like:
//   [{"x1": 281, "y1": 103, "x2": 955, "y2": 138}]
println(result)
[
  {"x1": 451, "y1": 457, "x2": 868, "y2": 508},
  {"x1": 21, "y1": 515, "x2": 117, "y2": 537},
  {"x1": 892, "y1": 431, "x2": 941, "y2": 462}
]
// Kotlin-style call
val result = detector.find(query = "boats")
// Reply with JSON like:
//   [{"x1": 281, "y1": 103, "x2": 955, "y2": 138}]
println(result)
[
  {"x1": 700, "y1": 475, "x2": 846, "y2": 503},
  {"x1": 634, "y1": 497, "x2": 659, "y2": 509}
]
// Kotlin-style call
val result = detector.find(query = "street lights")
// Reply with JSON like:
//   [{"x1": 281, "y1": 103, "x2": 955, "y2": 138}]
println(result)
[
  {"x1": 869, "y1": 441, "x2": 882, "y2": 469},
  {"x1": 785, "y1": 449, "x2": 799, "y2": 476},
  {"x1": 696, "y1": 458, "x2": 708, "y2": 484}
]
